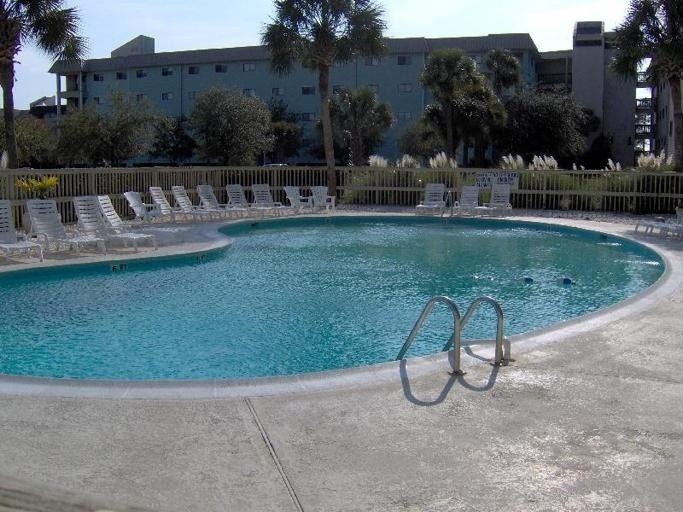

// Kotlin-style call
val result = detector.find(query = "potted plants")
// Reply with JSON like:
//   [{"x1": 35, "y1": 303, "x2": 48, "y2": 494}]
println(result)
[{"x1": 13, "y1": 174, "x2": 60, "y2": 237}]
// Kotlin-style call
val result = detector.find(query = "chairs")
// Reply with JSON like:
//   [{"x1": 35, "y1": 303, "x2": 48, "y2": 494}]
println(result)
[
  {"x1": 662, "y1": 207, "x2": 683, "y2": 241},
  {"x1": 413, "y1": 182, "x2": 512, "y2": 217}
]
